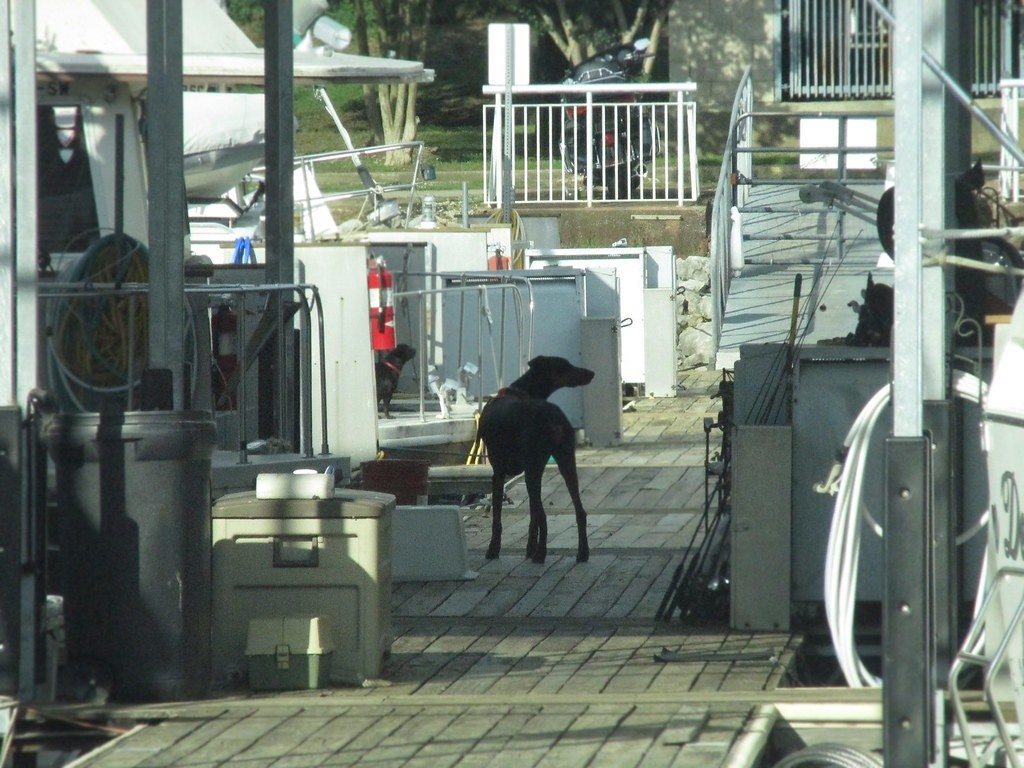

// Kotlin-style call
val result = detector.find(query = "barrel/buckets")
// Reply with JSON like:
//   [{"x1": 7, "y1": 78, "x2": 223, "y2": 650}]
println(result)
[{"x1": 363, "y1": 460, "x2": 431, "y2": 505}]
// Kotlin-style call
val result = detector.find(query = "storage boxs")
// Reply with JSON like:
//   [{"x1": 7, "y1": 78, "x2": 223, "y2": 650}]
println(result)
[{"x1": 244, "y1": 613, "x2": 335, "y2": 691}]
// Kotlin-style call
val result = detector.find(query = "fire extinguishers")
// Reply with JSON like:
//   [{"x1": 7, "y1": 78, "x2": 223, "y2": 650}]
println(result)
[{"x1": 368, "y1": 257, "x2": 397, "y2": 351}]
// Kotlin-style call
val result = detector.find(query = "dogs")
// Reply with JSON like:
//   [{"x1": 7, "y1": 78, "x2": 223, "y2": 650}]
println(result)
[
  {"x1": 478, "y1": 355, "x2": 596, "y2": 564},
  {"x1": 375, "y1": 344, "x2": 416, "y2": 419}
]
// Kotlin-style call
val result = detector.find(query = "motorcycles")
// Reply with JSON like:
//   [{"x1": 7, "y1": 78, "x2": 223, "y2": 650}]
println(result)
[{"x1": 557, "y1": 38, "x2": 661, "y2": 200}]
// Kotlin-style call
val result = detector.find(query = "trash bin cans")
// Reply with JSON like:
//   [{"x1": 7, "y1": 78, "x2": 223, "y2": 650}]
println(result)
[{"x1": 40, "y1": 407, "x2": 219, "y2": 701}]
